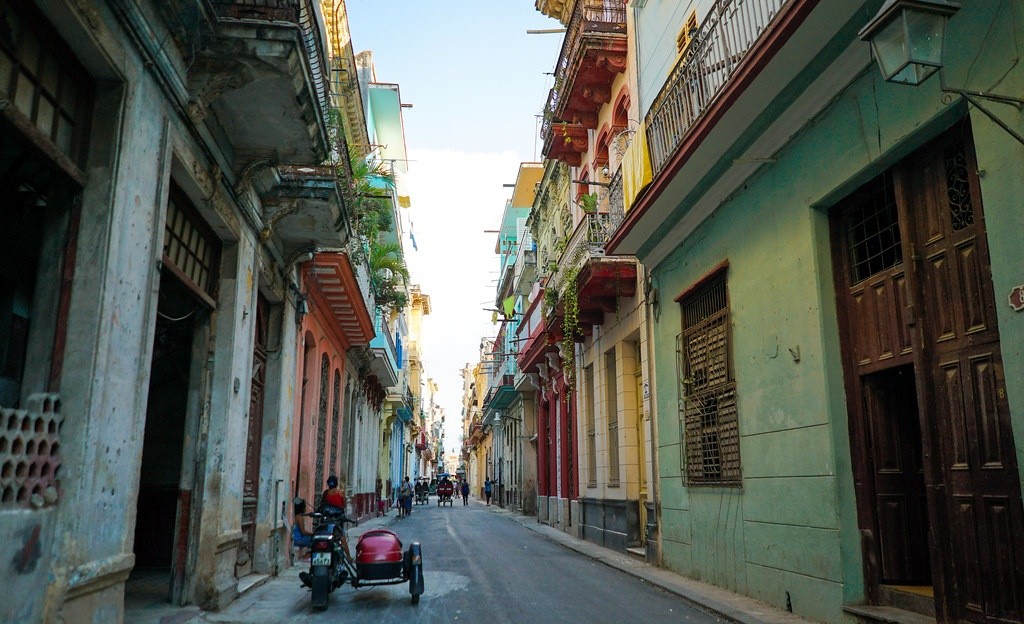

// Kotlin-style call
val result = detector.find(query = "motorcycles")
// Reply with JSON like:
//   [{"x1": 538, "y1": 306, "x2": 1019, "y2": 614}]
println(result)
[{"x1": 288, "y1": 475, "x2": 426, "y2": 612}]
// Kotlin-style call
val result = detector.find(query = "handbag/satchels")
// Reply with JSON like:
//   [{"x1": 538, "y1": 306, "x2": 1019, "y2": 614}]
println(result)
[{"x1": 410, "y1": 491, "x2": 414, "y2": 497}]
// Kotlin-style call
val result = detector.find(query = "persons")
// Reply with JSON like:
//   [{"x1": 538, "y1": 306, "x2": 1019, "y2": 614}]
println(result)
[
  {"x1": 415, "y1": 482, "x2": 429, "y2": 496},
  {"x1": 462, "y1": 479, "x2": 470, "y2": 506},
  {"x1": 377, "y1": 484, "x2": 388, "y2": 516},
  {"x1": 485, "y1": 477, "x2": 494, "y2": 506},
  {"x1": 397, "y1": 477, "x2": 413, "y2": 516},
  {"x1": 294, "y1": 475, "x2": 354, "y2": 561},
  {"x1": 430, "y1": 477, "x2": 461, "y2": 499}
]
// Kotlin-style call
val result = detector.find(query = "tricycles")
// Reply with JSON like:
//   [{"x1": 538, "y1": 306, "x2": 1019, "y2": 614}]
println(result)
[
  {"x1": 436, "y1": 473, "x2": 453, "y2": 507},
  {"x1": 413, "y1": 477, "x2": 430, "y2": 505}
]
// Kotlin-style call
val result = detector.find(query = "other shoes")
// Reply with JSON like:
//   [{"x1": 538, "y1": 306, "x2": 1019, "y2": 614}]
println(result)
[{"x1": 396, "y1": 515, "x2": 401, "y2": 517}]
